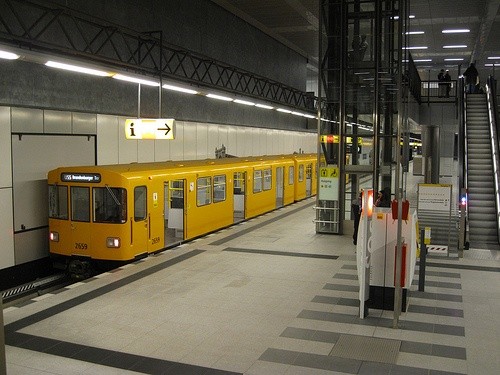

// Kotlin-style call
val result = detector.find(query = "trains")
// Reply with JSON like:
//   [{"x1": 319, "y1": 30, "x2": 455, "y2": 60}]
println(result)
[{"x1": 47, "y1": 152, "x2": 351, "y2": 280}]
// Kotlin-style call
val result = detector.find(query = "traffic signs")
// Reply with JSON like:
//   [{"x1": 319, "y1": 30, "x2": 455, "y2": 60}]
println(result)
[{"x1": 124, "y1": 117, "x2": 176, "y2": 140}]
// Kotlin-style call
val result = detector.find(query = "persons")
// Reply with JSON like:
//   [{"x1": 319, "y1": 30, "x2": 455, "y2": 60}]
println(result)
[
  {"x1": 444, "y1": 70, "x2": 451, "y2": 97},
  {"x1": 438, "y1": 69, "x2": 444, "y2": 87},
  {"x1": 463, "y1": 63, "x2": 478, "y2": 94},
  {"x1": 376, "y1": 190, "x2": 388, "y2": 207},
  {"x1": 358, "y1": 34, "x2": 368, "y2": 61},
  {"x1": 352, "y1": 194, "x2": 362, "y2": 244}
]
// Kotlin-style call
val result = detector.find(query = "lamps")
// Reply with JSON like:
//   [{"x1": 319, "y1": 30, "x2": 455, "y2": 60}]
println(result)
[{"x1": 45, "y1": 61, "x2": 317, "y2": 118}]
